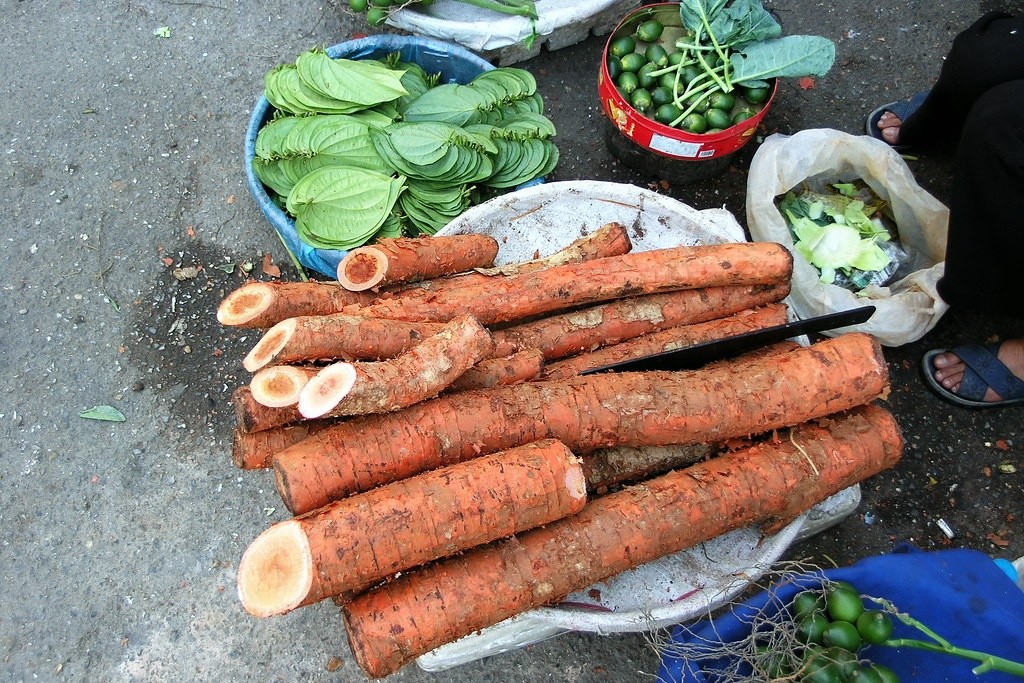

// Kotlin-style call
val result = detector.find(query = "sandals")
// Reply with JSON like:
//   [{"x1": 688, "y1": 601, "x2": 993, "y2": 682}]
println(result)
[
  {"x1": 924, "y1": 340, "x2": 1024, "y2": 408},
  {"x1": 865, "y1": 90, "x2": 932, "y2": 151}
]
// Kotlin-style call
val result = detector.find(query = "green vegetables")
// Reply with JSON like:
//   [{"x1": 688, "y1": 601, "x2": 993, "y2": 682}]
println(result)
[
  {"x1": 255, "y1": 49, "x2": 556, "y2": 250},
  {"x1": 646, "y1": 1, "x2": 835, "y2": 127}
]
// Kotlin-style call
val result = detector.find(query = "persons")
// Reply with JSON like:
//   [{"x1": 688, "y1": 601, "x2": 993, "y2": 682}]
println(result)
[{"x1": 863, "y1": 11, "x2": 1023, "y2": 408}]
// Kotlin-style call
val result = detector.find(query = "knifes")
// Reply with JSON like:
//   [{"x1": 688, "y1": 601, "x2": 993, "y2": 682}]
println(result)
[{"x1": 580, "y1": 305, "x2": 877, "y2": 375}]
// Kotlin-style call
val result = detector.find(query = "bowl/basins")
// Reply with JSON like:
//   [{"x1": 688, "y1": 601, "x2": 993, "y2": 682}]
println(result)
[{"x1": 245, "y1": 33, "x2": 546, "y2": 276}]
